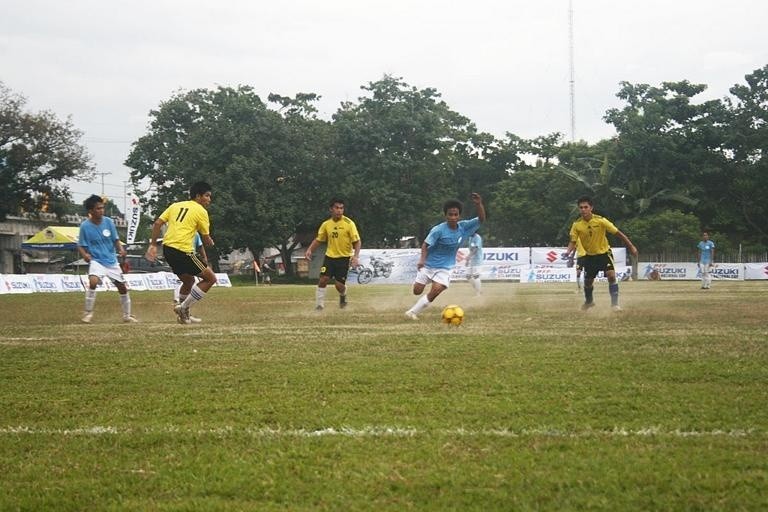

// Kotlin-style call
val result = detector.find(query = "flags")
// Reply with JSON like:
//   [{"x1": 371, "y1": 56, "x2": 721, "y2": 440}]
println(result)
[{"x1": 253, "y1": 260, "x2": 260, "y2": 273}]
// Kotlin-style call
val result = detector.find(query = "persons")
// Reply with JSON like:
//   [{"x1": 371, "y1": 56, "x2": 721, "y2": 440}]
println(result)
[
  {"x1": 262, "y1": 260, "x2": 275, "y2": 286},
  {"x1": 465, "y1": 232, "x2": 481, "y2": 295},
  {"x1": 78, "y1": 194, "x2": 138, "y2": 322},
  {"x1": 696, "y1": 263, "x2": 701, "y2": 278},
  {"x1": 562, "y1": 196, "x2": 637, "y2": 310},
  {"x1": 567, "y1": 236, "x2": 595, "y2": 293},
  {"x1": 621, "y1": 268, "x2": 631, "y2": 280},
  {"x1": 406, "y1": 192, "x2": 485, "y2": 319},
  {"x1": 306, "y1": 197, "x2": 361, "y2": 309},
  {"x1": 649, "y1": 268, "x2": 661, "y2": 280},
  {"x1": 146, "y1": 182, "x2": 218, "y2": 323},
  {"x1": 697, "y1": 232, "x2": 715, "y2": 289}
]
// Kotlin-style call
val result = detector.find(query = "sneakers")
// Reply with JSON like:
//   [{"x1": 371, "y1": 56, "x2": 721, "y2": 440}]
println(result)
[
  {"x1": 612, "y1": 306, "x2": 623, "y2": 312},
  {"x1": 123, "y1": 314, "x2": 137, "y2": 323},
  {"x1": 174, "y1": 304, "x2": 201, "y2": 324},
  {"x1": 582, "y1": 303, "x2": 595, "y2": 310}
]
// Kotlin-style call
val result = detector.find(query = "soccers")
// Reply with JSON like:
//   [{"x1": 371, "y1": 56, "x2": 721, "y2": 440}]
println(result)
[{"x1": 443, "y1": 305, "x2": 464, "y2": 326}]
